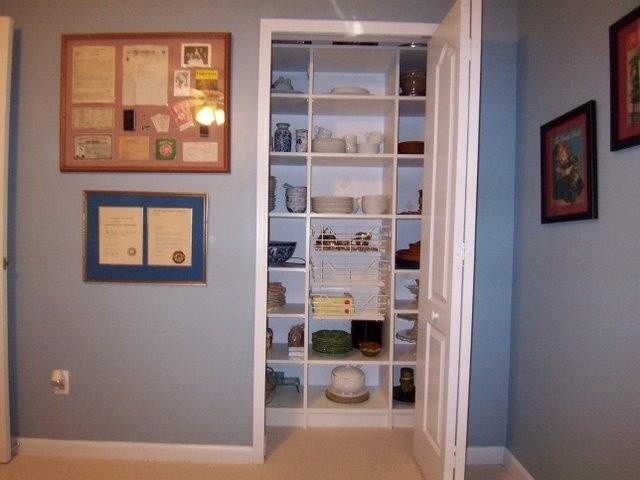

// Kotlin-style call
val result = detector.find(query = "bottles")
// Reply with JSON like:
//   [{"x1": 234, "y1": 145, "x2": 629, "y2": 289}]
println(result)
[{"x1": 294, "y1": 128, "x2": 309, "y2": 153}]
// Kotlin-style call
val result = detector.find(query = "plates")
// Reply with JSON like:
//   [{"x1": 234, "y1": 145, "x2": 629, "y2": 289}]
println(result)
[
  {"x1": 270, "y1": 88, "x2": 304, "y2": 94},
  {"x1": 332, "y1": 85, "x2": 370, "y2": 95},
  {"x1": 311, "y1": 329, "x2": 354, "y2": 358},
  {"x1": 393, "y1": 384, "x2": 415, "y2": 403},
  {"x1": 310, "y1": 195, "x2": 388, "y2": 214},
  {"x1": 328, "y1": 385, "x2": 369, "y2": 398},
  {"x1": 311, "y1": 125, "x2": 386, "y2": 155}
]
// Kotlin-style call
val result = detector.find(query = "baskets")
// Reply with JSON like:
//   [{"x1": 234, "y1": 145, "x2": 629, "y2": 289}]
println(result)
[
  {"x1": 312, "y1": 329, "x2": 353, "y2": 359},
  {"x1": 267, "y1": 282, "x2": 286, "y2": 313}
]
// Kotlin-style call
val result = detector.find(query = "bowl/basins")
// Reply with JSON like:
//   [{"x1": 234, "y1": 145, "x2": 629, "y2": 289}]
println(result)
[
  {"x1": 399, "y1": 140, "x2": 424, "y2": 154},
  {"x1": 330, "y1": 363, "x2": 367, "y2": 394},
  {"x1": 400, "y1": 69, "x2": 426, "y2": 96},
  {"x1": 360, "y1": 347, "x2": 382, "y2": 357},
  {"x1": 268, "y1": 240, "x2": 298, "y2": 268},
  {"x1": 284, "y1": 186, "x2": 307, "y2": 212}
]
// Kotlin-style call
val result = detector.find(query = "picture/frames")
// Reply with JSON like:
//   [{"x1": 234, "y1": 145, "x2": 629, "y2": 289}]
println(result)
[
  {"x1": 59, "y1": 32, "x2": 232, "y2": 173},
  {"x1": 539, "y1": 8, "x2": 640, "y2": 226},
  {"x1": 79, "y1": 189, "x2": 208, "y2": 286}
]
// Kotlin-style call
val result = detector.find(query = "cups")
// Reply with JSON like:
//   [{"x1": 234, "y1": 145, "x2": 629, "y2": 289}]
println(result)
[
  {"x1": 399, "y1": 367, "x2": 414, "y2": 394},
  {"x1": 273, "y1": 371, "x2": 284, "y2": 385}
]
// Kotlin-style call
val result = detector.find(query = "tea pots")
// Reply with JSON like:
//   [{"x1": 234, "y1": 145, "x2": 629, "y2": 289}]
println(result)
[{"x1": 274, "y1": 76, "x2": 294, "y2": 89}]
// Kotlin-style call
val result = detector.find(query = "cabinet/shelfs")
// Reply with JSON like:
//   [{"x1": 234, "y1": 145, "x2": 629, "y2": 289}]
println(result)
[{"x1": 264, "y1": 44, "x2": 428, "y2": 432}]
